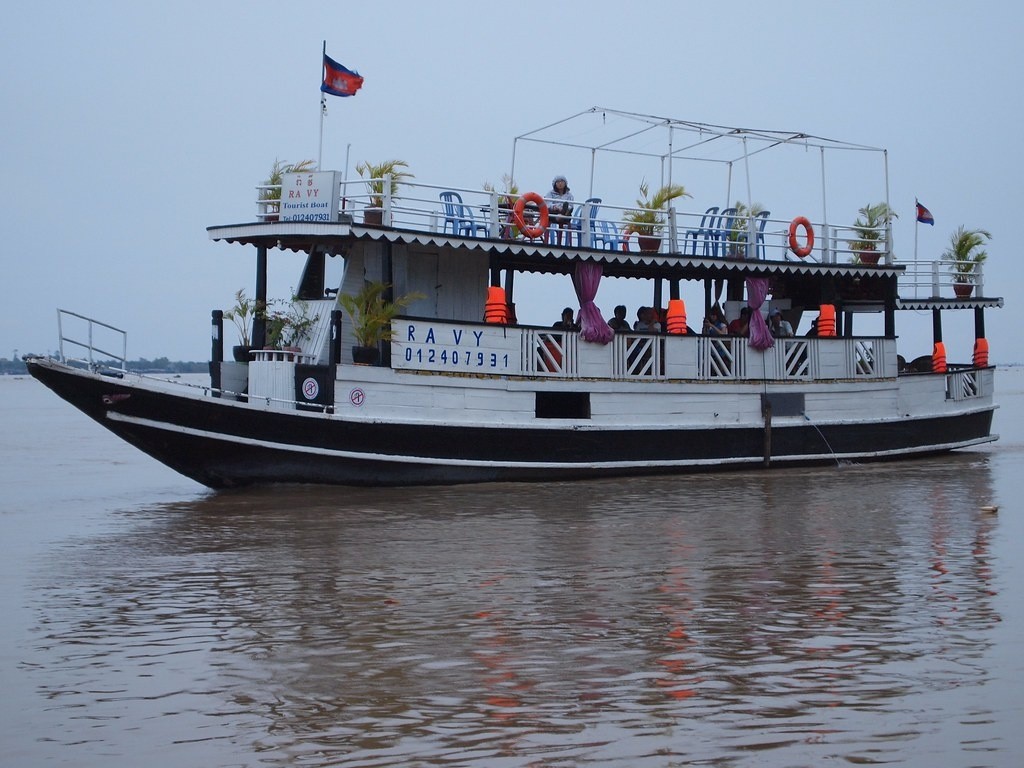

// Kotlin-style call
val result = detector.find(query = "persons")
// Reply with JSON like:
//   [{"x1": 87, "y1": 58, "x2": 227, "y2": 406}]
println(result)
[
  {"x1": 729, "y1": 306, "x2": 750, "y2": 335},
  {"x1": 608, "y1": 305, "x2": 633, "y2": 347},
  {"x1": 767, "y1": 309, "x2": 794, "y2": 351},
  {"x1": 703, "y1": 311, "x2": 727, "y2": 358},
  {"x1": 364, "y1": 267, "x2": 376, "y2": 314},
  {"x1": 542, "y1": 175, "x2": 574, "y2": 224},
  {"x1": 634, "y1": 304, "x2": 661, "y2": 370},
  {"x1": 545, "y1": 307, "x2": 576, "y2": 340}
]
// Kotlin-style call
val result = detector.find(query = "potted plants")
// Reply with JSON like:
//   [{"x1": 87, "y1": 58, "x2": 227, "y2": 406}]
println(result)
[
  {"x1": 485, "y1": 173, "x2": 523, "y2": 240},
  {"x1": 355, "y1": 160, "x2": 416, "y2": 227},
  {"x1": 849, "y1": 202, "x2": 898, "y2": 263},
  {"x1": 624, "y1": 178, "x2": 694, "y2": 252},
  {"x1": 939, "y1": 224, "x2": 991, "y2": 296},
  {"x1": 336, "y1": 283, "x2": 428, "y2": 366},
  {"x1": 729, "y1": 200, "x2": 762, "y2": 259},
  {"x1": 258, "y1": 158, "x2": 318, "y2": 221},
  {"x1": 221, "y1": 287, "x2": 319, "y2": 359}
]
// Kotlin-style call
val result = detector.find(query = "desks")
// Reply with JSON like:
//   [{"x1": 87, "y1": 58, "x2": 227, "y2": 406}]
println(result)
[{"x1": 480, "y1": 203, "x2": 558, "y2": 242}]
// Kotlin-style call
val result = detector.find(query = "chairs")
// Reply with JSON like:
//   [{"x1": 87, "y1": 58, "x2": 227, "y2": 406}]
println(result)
[
  {"x1": 439, "y1": 190, "x2": 631, "y2": 252},
  {"x1": 685, "y1": 207, "x2": 771, "y2": 259}
]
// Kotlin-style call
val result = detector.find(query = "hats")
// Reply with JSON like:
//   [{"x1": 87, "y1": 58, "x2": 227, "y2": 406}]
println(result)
[{"x1": 552, "y1": 175, "x2": 568, "y2": 188}]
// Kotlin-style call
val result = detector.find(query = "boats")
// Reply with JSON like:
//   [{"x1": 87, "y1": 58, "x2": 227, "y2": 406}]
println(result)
[{"x1": 20, "y1": 36, "x2": 1006, "y2": 497}]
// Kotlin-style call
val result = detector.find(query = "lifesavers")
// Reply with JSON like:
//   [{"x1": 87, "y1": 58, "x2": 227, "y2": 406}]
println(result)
[
  {"x1": 623, "y1": 229, "x2": 644, "y2": 252},
  {"x1": 514, "y1": 192, "x2": 550, "y2": 238},
  {"x1": 789, "y1": 216, "x2": 815, "y2": 258}
]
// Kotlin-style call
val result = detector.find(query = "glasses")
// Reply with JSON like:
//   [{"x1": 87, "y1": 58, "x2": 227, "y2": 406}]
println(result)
[{"x1": 556, "y1": 180, "x2": 564, "y2": 184}]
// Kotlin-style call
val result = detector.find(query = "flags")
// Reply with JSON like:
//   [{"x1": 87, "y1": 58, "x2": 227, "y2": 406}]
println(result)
[
  {"x1": 915, "y1": 201, "x2": 934, "y2": 226},
  {"x1": 321, "y1": 53, "x2": 364, "y2": 98}
]
